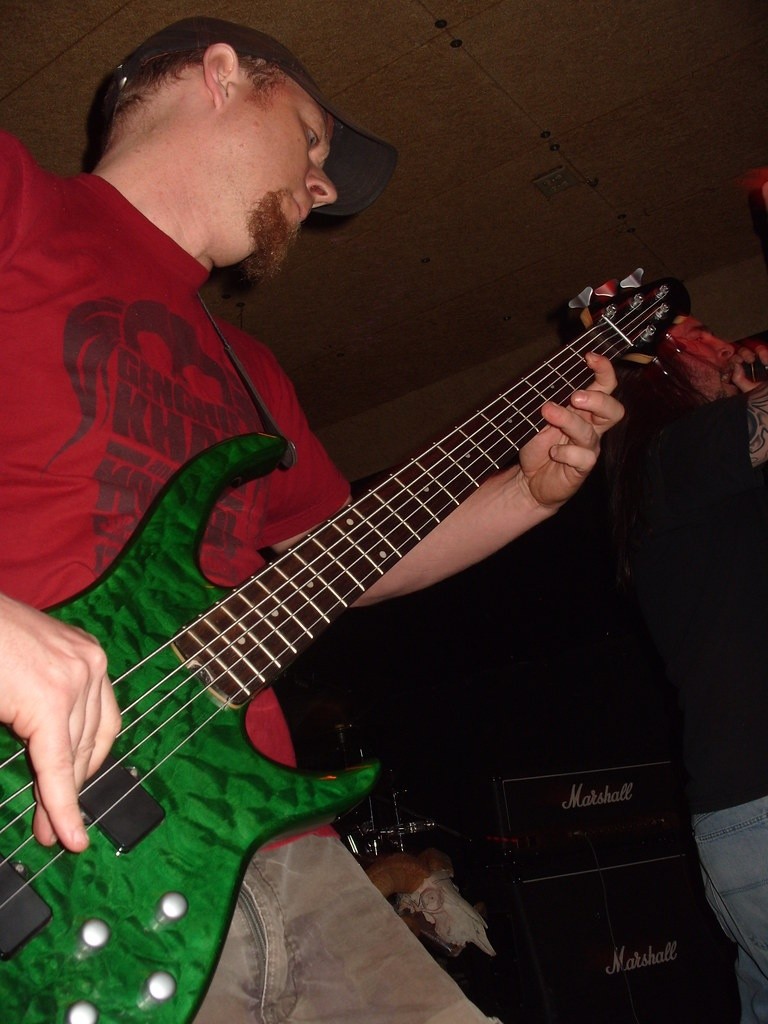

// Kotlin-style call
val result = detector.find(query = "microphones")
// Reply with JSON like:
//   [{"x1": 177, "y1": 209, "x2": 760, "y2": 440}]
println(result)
[
  {"x1": 335, "y1": 721, "x2": 346, "y2": 751},
  {"x1": 721, "y1": 357, "x2": 768, "y2": 383}
]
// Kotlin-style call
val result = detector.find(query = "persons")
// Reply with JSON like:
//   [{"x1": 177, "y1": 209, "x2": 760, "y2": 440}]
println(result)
[
  {"x1": 0, "y1": 14, "x2": 625, "y2": 1024},
  {"x1": 549, "y1": 278, "x2": 768, "y2": 1024}
]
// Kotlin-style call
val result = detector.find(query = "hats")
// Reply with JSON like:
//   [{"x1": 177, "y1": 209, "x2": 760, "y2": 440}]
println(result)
[
  {"x1": 93, "y1": 15, "x2": 398, "y2": 216},
  {"x1": 547, "y1": 268, "x2": 692, "y2": 367}
]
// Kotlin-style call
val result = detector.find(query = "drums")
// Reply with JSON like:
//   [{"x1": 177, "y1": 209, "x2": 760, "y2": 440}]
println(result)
[{"x1": 389, "y1": 819, "x2": 440, "y2": 853}]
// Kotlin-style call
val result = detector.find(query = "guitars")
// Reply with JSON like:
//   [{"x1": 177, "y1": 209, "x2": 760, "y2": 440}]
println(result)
[{"x1": 1, "y1": 267, "x2": 693, "y2": 1024}]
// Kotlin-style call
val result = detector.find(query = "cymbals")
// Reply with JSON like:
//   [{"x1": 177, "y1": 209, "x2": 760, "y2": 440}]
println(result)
[{"x1": 315, "y1": 722, "x2": 372, "y2": 741}]
